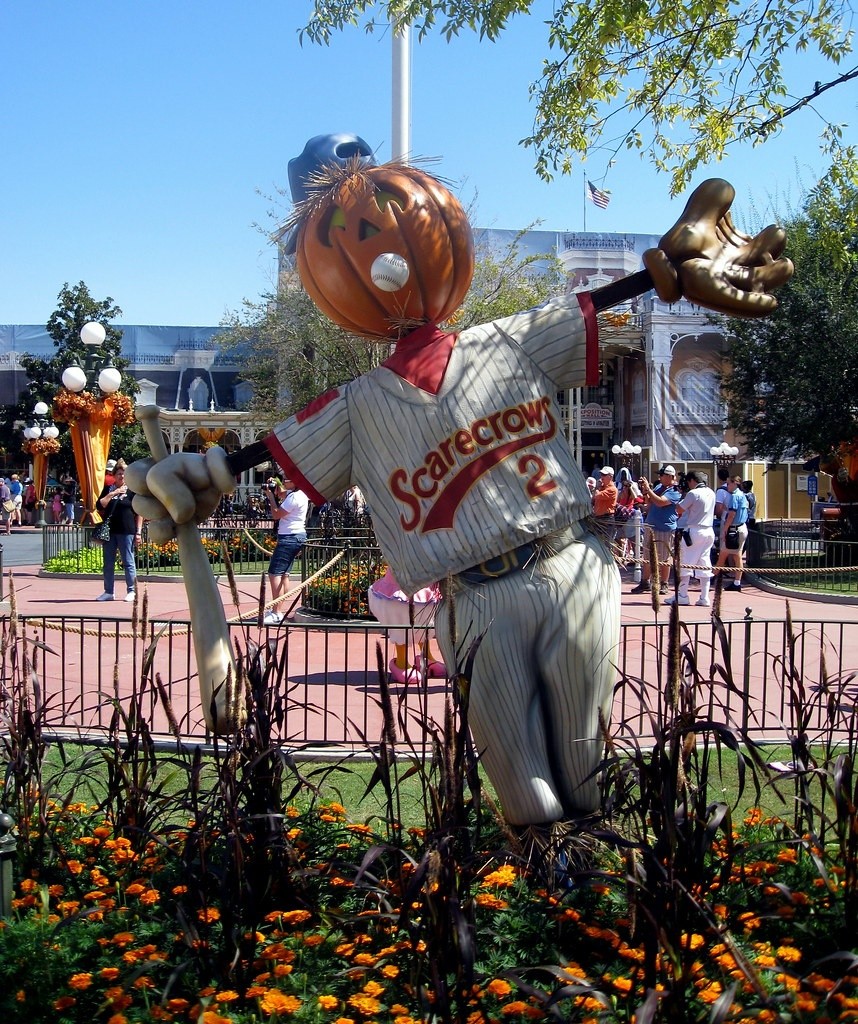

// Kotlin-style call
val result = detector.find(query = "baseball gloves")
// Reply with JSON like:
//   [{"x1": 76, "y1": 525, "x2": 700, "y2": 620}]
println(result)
[{"x1": 641, "y1": 175, "x2": 795, "y2": 318}]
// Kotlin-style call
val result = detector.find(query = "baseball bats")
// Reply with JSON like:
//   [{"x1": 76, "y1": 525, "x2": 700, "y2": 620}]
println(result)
[{"x1": 134, "y1": 403, "x2": 249, "y2": 734}]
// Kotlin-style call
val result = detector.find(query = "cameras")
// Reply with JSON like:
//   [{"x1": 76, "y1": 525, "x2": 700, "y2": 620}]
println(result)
[
  {"x1": 638, "y1": 479, "x2": 644, "y2": 484},
  {"x1": 672, "y1": 472, "x2": 691, "y2": 493},
  {"x1": 261, "y1": 480, "x2": 277, "y2": 494}
]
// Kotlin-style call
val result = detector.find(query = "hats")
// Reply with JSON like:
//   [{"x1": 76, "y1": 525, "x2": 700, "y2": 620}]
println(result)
[
  {"x1": 65, "y1": 476, "x2": 75, "y2": 481},
  {"x1": 599, "y1": 466, "x2": 614, "y2": 475},
  {"x1": 655, "y1": 465, "x2": 676, "y2": 476},
  {"x1": 106, "y1": 459, "x2": 117, "y2": 471}
]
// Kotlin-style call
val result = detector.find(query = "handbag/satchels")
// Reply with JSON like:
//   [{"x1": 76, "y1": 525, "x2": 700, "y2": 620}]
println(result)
[
  {"x1": 614, "y1": 503, "x2": 634, "y2": 528},
  {"x1": 725, "y1": 531, "x2": 739, "y2": 549},
  {"x1": 91, "y1": 519, "x2": 110, "y2": 545},
  {"x1": 2, "y1": 499, "x2": 16, "y2": 513}
]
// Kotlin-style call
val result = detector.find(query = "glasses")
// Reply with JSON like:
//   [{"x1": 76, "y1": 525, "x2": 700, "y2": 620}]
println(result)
[{"x1": 282, "y1": 478, "x2": 291, "y2": 483}]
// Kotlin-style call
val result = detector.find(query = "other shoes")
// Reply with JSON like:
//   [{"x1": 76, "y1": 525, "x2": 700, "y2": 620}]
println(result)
[
  {"x1": 1, "y1": 533, "x2": 11, "y2": 536},
  {"x1": 18, "y1": 523, "x2": 22, "y2": 527}
]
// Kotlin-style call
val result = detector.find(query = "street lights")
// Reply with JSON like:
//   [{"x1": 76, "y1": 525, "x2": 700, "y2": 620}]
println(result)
[
  {"x1": 709, "y1": 441, "x2": 739, "y2": 483},
  {"x1": 62, "y1": 322, "x2": 122, "y2": 549},
  {"x1": 611, "y1": 441, "x2": 642, "y2": 479},
  {"x1": 20, "y1": 400, "x2": 61, "y2": 529}
]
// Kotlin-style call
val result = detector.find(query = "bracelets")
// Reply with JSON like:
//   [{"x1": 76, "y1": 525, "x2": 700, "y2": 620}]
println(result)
[
  {"x1": 137, "y1": 528, "x2": 141, "y2": 534},
  {"x1": 645, "y1": 487, "x2": 650, "y2": 491}
]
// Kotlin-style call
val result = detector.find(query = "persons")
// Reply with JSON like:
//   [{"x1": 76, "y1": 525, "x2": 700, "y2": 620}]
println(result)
[
  {"x1": 124, "y1": 132, "x2": 794, "y2": 889},
  {"x1": 631, "y1": 465, "x2": 682, "y2": 596},
  {"x1": 10, "y1": 474, "x2": 22, "y2": 527},
  {"x1": 95, "y1": 458, "x2": 143, "y2": 602},
  {"x1": 585, "y1": 463, "x2": 644, "y2": 558},
  {"x1": 714, "y1": 469, "x2": 730, "y2": 550},
  {"x1": 104, "y1": 459, "x2": 116, "y2": 485},
  {"x1": 305, "y1": 501, "x2": 330, "y2": 539},
  {"x1": 742, "y1": 481, "x2": 756, "y2": 526},
  {"x1": 712, "y1": 474, "x2": 748, "y2": 592},
  {"x1": 18, "y1": 470, "x2": 36, "y2": 526},
  {"x1": 255, "y1": 474, "x2": 308, "y2": 624},
  {"x1": 664, "y1": 471, "x2": 715, "y2": 606},
  {"x1": 49, "y1": 486, "x2": 61, "y2": 524},
  {"x1": 62, "y1": 477, "x2": 76, "y2": 524},
  {"x1": 0, "y1": 478, "x2": 11, "y2": 536},
  {"x1": 345, "y1": 485, "x2": 363, "y2": 524},
  {"x1": 2, "y1": 473, "x2": 11, "y2": 484}
]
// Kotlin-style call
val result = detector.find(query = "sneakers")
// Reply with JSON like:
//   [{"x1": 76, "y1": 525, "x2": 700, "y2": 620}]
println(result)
[
  {"x1": 631, "y1": 580, "x2": 652, "y2": 594},
  {"x1": 125, "y1": 591, "x2": 135, "y2": 602},
  {"x1": 255, "y1": 609, "x2": 284, "y2": 624},
  {"x1": 664, "y1": 593, "x2": 690, "y2": 604},
  {"x1": 96, "y1": 593, "x2": 115, "y2": 601},
  {"x1": 724, "y1": 582, "x2": 741, "y2": 592},
  {"x1": 660, "y1": 581, "x2": 669, "y2": 594},
  {"x1": 697, "y1": 576, "x2": 715, "y2": 585},
  {"x1": 695, "y1": 595, "x2": 710, "y2": 607}
]
